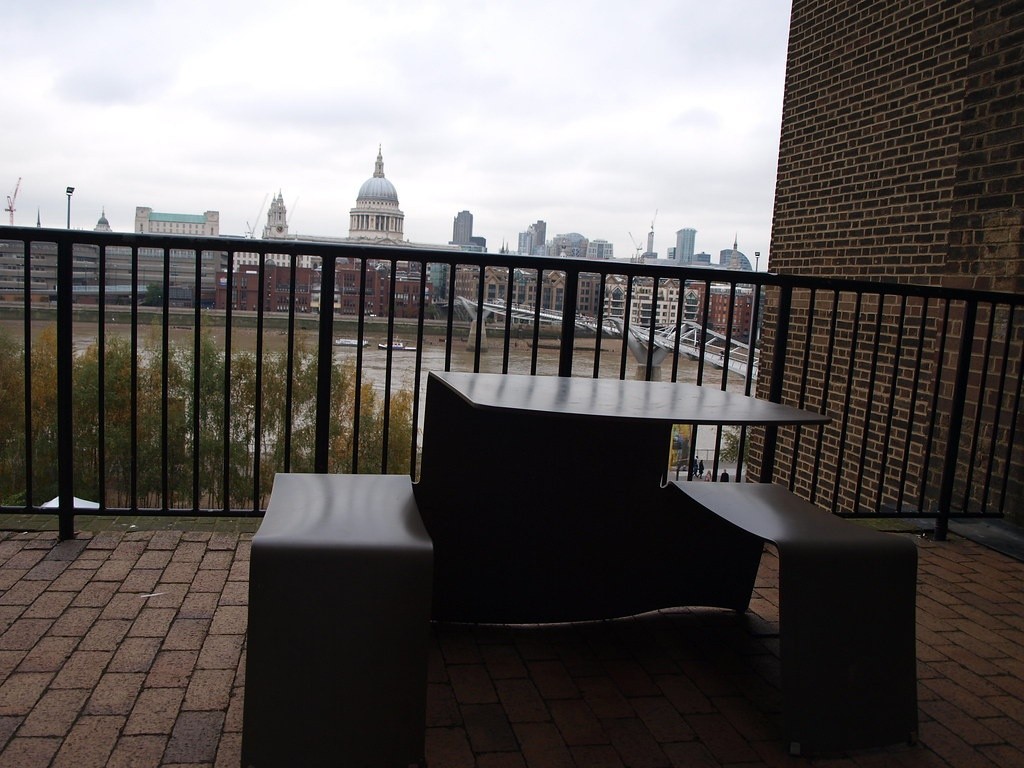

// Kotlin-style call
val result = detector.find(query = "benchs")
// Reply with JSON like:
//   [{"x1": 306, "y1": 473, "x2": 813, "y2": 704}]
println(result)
[
  {"x1": 239, "y1": 470, "x2": 432, "y2": 767},
  {"x1": 666, "y1": 478, "x2": 919, "y2": 746}
]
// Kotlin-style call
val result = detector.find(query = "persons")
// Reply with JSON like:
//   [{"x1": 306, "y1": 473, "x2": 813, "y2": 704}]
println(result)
[
  {"x1": 697, "y1": 460, "x2": 704, "y2": 479},
  {"x1": 720, "y1": 469, "x2": 729, "y2": 482},
  {"x1": 693, "y1": 456, "x2": 699, "y2": 477},
  {"x1": 705, "y1": 470, "x2": 711, "y2": 482}
]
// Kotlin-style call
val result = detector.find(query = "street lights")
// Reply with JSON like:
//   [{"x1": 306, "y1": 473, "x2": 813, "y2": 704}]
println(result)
[
  {"x1": 754, "y1": 252, "x2": 760, "y2": 272},
  {"x1": 65, "y1": 187, "x2": 75, "y2": 229}
]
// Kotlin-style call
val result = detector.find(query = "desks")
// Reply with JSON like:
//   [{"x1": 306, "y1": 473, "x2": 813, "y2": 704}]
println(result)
[{"x1": 420, "y1": 369, "x2": 830, "y2": 626}]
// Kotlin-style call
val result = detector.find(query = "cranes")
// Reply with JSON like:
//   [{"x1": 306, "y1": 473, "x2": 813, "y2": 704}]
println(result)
[
  {"x1": 5, "y1": 176, "x2": 21, "y2": 226},
  {"x1": 629, "y1": 232, "x2": 643, "y2": 253},
  {"x1": 651, "y1": 208, "x2": 658, "y2": 231},
  {"x1": 246, "y1": 190, "x2": 269, "y2": 236}
]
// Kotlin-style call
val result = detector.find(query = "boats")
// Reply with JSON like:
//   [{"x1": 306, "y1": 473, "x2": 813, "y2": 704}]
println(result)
[
  {"x1": 378, "y1": 336, "x2": 416, "y2": 351},
  {"x1": 333, "y1": 339, "x2": 371, "y2": 348},
  {"x1": 526, "y1": 342, "x2": 609, "y2": 351}
]
